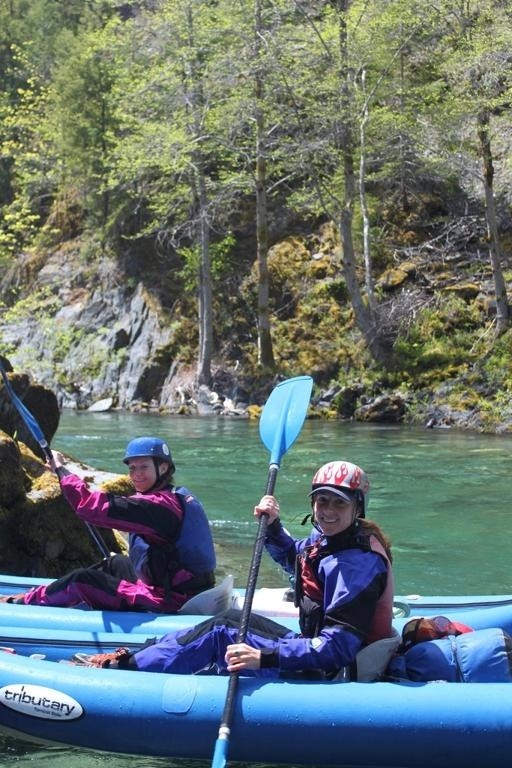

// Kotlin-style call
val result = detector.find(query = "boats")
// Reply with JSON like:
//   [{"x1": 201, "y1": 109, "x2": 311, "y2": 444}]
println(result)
[
  {"x1": 0, "y1": 627, "x2": 512, "y2": 763},
  {"x1": 0, "y1": 574, "x2": 511, "y2": 635}
]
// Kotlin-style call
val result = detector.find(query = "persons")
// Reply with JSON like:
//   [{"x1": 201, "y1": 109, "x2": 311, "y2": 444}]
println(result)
[
  {"x1": 72, "y1": 461, "x2": 396, "y2": 681},
  {"x1": 0, "y1": 437, "x2": 216, "y2": 613}
]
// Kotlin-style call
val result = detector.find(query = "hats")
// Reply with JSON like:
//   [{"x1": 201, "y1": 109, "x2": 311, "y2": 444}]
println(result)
[{"x1": 308, "y1": 485, "x2": 354, "y2": 504}]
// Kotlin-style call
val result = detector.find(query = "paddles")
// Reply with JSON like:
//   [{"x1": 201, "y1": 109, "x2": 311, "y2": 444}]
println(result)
[
  {"x1": 2, "y1": 372, "x2": 112, "y2": 561},
  {"x1": 211, "y1": 376, "x2": 313, "y2": 768}
]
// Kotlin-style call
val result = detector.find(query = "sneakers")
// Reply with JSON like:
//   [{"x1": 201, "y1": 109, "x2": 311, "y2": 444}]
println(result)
[{"x1": 73, "y1": 646, "x2": 128, "y2": 668}]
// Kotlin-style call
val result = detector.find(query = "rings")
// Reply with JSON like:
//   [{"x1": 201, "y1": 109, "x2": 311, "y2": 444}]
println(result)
[{"x1": 233, "y1": 649, "x2": 239, "y2": 655}]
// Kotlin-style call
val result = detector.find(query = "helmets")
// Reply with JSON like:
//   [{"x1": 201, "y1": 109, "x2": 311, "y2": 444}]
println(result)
[
  {"x1": 310, "y1": 461, "x2": 369, "y2": 518},
  {"x1": 123, "y1": 436, "x2": 173, "y2": 466}
]
